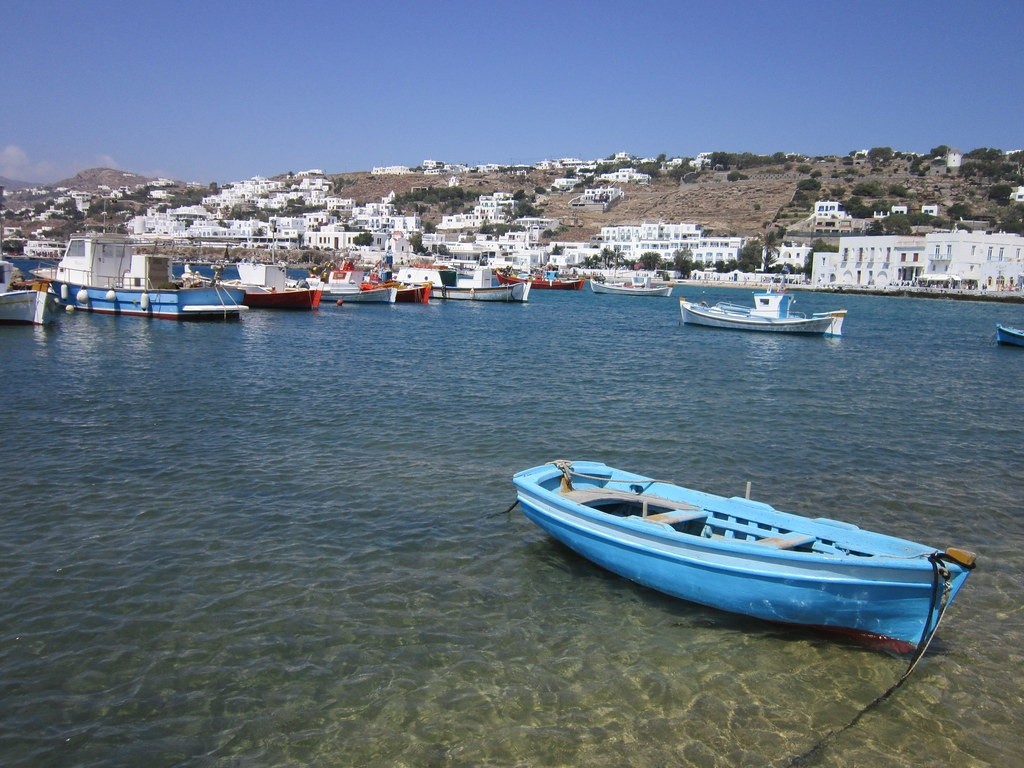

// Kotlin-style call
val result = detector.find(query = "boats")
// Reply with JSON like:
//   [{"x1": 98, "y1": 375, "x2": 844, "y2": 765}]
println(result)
[
  {"x1": 27, "y1": 212, "x2": 251, "y2": 321},
  {"x1": 589, "y1": 274, "x2": 673, "y2": 298},
  {"x1": 512, "y1": 457, "x2": 977, "y2": 664},
  {"x1": 996, "y1": 321, "x2": 1024, "y2": 348},
  {"x1": 177, "y1": 243, "x2": 585, "y2": 313},
  {"x1": 678, "y1": 272, "x2": 847, "y2": 335},
  {"x1": 0, "y1": 260, "x2": 61, "y2": 325}
]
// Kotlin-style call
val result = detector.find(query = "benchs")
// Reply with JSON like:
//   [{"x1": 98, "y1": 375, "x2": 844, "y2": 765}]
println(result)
[
  {"x1": 644, "y1": 510, "x2": 705, "y2": 525},
  {"x1": 753, "y1": 533, "x2": 815, "y2": 549}
]
